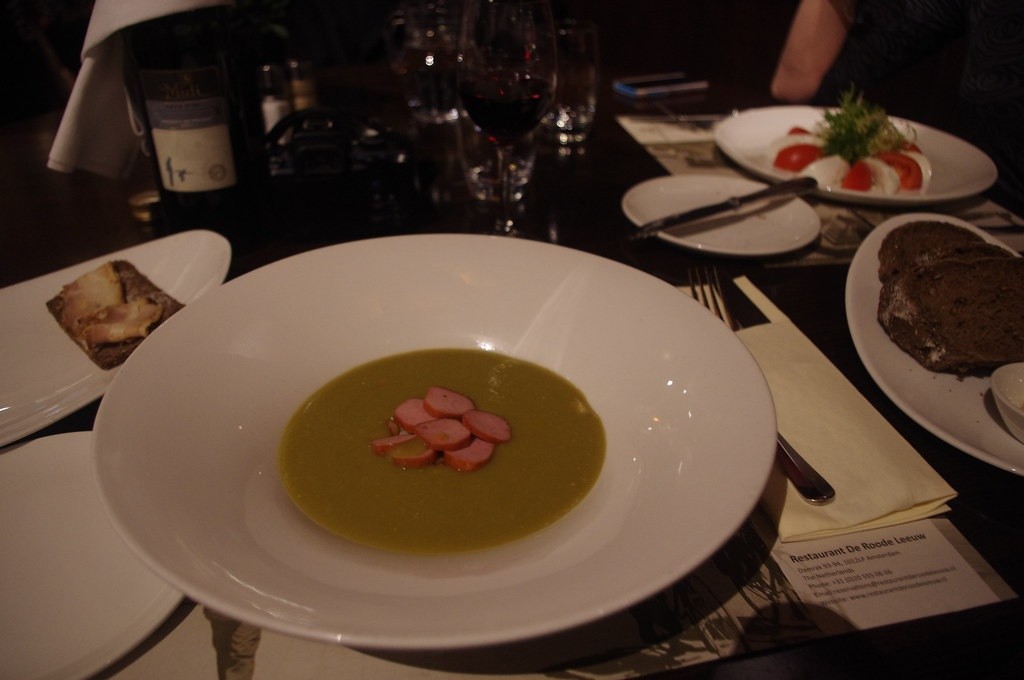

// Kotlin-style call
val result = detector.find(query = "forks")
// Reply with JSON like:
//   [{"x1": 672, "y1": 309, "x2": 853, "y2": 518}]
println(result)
[
  {"x1": 849, "y1": 209, "x2": 1024, "y2": 234},
  {"x1": 687, "y1": 266, "x2": 835, "y2": 501}
]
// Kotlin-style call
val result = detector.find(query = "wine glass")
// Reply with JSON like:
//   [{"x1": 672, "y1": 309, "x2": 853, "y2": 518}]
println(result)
[{"x1": 457, "y1": 0, "x2": 557, "y2": 239}]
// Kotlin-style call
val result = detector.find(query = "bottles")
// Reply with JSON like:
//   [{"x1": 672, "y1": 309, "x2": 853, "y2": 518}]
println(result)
[
  {"x1": 260, "y1": 64, "x2": 291, "y2": 145},
  {"x1": 123, "y1": 7, "x2": 268, "y2": 245}
]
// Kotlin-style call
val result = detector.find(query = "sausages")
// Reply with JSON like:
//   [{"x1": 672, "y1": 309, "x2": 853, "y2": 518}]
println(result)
[{"x1": 372, "y1": 388, "x2": 512, "y2": 470}]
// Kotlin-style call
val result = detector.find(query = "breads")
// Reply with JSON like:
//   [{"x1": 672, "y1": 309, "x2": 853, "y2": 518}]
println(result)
[
  {"x1": 45, "y1": 260, "x2": 186, "y2": 369},
  {"x1": 876, "y1": 220, "x2": 1024, "y2": 371}
]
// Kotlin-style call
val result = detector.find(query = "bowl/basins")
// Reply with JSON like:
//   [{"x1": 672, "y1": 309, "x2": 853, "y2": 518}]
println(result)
[
  {"x1": 91, "y1": 235, "x2": 775, "y2": 650},
  {"x1": 990, "y1": 362, "x2": 1024, "y2": 444}
]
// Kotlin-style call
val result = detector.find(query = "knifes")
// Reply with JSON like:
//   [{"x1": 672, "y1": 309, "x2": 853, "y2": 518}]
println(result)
[
  {"x1": 635, "y1": 177, "x2": 817, "y2": 237},
  {"x1": 720, "y1": 269, "x2": 771, "y2": 328}
]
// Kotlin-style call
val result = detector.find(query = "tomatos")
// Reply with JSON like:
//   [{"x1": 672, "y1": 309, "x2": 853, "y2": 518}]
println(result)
[
  {"x1": 771, "y1": 144, "x2": 823, "y2": 171},
  {"x1": 901, "y1": 139, "x2": 923, "y2": 155},
  {"x1": 840, "y1": 163, "x2": 872, "y2": 191},
  {"x1": 788, "y1": 127, "x2": 810, "y2": 136},
  {"x1": 879, "y1": 152, "x2": 922, "y2": 190}
]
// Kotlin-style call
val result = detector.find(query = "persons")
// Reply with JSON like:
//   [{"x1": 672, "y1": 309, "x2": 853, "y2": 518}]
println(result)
[{"x1": 772, "y1": 0, "x2": 1024, "y2": 223}]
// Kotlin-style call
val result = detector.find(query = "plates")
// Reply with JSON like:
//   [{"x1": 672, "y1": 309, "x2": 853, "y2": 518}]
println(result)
[
  {"x1": 713, "y1": 106, "x2": 997, "y2": 205},
  {"x1": 0, "y1": 432, "x2": 186, "y2": 680},
  {"x1": 0, "y1": 229, "x2": 231, "y2": 448},
  {"x1": 622, "y1": 174, "x2": 820, "y2": 256},
  {"x1": 845, "y1": 214, "x2": 1024, "y2": 476}
]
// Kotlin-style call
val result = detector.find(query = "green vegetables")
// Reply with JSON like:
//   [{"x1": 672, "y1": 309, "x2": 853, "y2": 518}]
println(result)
[{"x1": 814, "y1": 82, "x2": 904, "y2": 161}]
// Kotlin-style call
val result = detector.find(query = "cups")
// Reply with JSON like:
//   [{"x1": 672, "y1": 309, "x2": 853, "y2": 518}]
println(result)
[
  {"x1": 542, "y1": 19, "x2": 596, "y2": 143},
  {"x1": 384, "y1": 0, "x2": 465, "y2": 122},
  {"x1": 458, "y1": 96, "x2": 537, "y2": 202}
]
